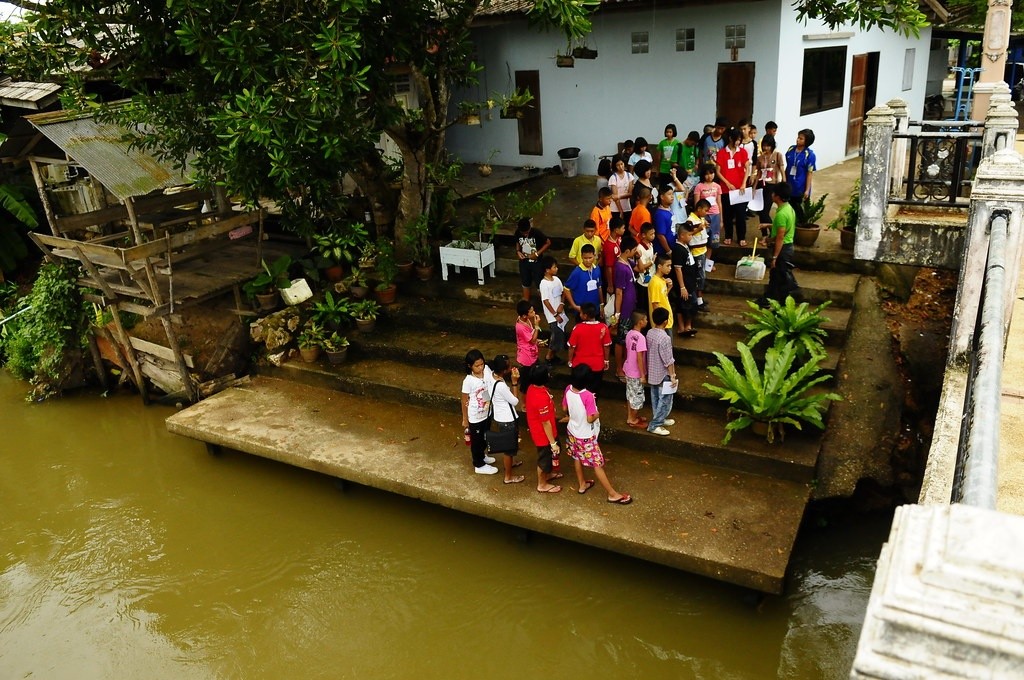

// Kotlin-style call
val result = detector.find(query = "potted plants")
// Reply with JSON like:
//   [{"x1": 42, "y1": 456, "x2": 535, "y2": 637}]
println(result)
[
  {"x1": 482, "y1": 100, "x2": 495, "y2": 120},
  {"x1": 548, "y1": 37, "x2": 602, "y2": 68},
  {"x1": 245, "y1": 157, "x2": 559, "y2": 362},
  {"x1": 454, "y1": 96, "x2": 481, "y2": 125},
  {"x1": 703, "y1": 350, "x2": 837, "y2": 444},
  {"x1": 793, "y1": 192, "x2": 827, "y2": 247},
  {"x1": 733, "y1": 300, "x2": 830, "y2": 358},
  {"x1": 831, "y1": 188, "x2": 862, "y2": 248},
  {"x1": 487, "y1": 86, "x2": 532, "y2": 120}
]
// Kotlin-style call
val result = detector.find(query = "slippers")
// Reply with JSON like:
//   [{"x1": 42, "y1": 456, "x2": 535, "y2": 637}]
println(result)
[
  {"x1": 545, "y1": 472, "x2": 564, "y2": 481},
  {"x1": 578, "y1": 480, "x2": 594, "y2": 494},
  {"x1": 606, "y1": 493, "x2": 632, "y2": 505},
  {"x1": 616, "y1": 372, "x2": 626, "y2": 383},
  {"x1": 504, "y1": 475, "x2": 525, "y2": 484},
  {"x1": 512, "y1": 460, "x2": 523, "y2": 467},
  {"x1": 626, "y1": 415, "x2": 648, "y2": 429},
  {"x1": 537, "y1": 483, "x2": 562, "y2": 493},
  {"x1": 544, "y1": 354, "x2": 553, "y2": 366}
]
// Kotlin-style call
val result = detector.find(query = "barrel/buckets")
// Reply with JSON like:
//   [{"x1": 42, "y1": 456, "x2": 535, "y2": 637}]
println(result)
[{"x1": 560, "y1": 158, "x2": 578, "y2": 178}]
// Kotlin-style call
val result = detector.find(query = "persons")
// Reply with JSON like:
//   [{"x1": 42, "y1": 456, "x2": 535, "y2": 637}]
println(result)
[
  {"x1": 656, "y1": 117, "x2": 817, "y2": 309},
  {"x1": 515, "y1": 217, "x2": 551, "y2": 301},
  {"x1": 590, "y1": 153, "x2": 723, "y2": 337},
  {"x1": 514, "y1": 300, "x2": 675, "y2": 435},
  {"x1": 461, "y1": 349, "x2": 633, "y2": 504},
  {"x1": 621, "y1": 137, "x2": 653, "y2": 185},
  {"x1": 563, "y1": 218, "x2": 674, "y2": 383}
]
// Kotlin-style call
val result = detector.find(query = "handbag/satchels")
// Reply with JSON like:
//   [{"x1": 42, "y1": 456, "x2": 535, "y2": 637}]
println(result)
[
  {"x1": 767, "y1": 237, "x2": 777, "y2": 248},
  {"x1": 603, "y1": 292, "x2": 615, "y2": 328},
  {"x1": 485, "y1": 431, "x2": 515, "y2": 453}
]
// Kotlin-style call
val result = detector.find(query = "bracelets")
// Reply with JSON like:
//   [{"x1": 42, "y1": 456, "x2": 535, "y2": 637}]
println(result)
[
  {"x1": 516, "y1": 250, "x2": 521, "y2": 254},
  {"x1": 534, "y1": 252, "x2": 538, "y2": 257},
  {"x1": 671, "y1": 374, "x2": 677, "y2": 378},
  {"x1": 681, "y1": 286, "x2": 686, "y2": 290},
  {"x1": 551, "y1": 441, "x2": 557, "y2": 446},
  {"x1": 773, "y1": 256, "x2": 777, "y2": 259},
  {"x1": 666, "y1": 249, "x2": 672, "y2": 256},
  {"x1": 615, "y1": 312, "x2": 620, "y2": 320},
  {"x1": 651, "y1": 261, "x2": 655, "y2": 265},
  {"x1": 512, "y1": 383, "x2": 518, "y2": 386},
  {"x1": 553, "y1": 312, "x2": 558, "y2": 317},
  {"x1": 674, "y1": 177, "x2": 678, "y2": 181},
  {"x1": 604, "y1": 360, "x2": 610, "y2": 362},
  {"x1": 535, "y1": 325, "x2": 542, "y2": 331},
  {"x1": 636, "y1": 232, "x2": 639, "y2": 237}
]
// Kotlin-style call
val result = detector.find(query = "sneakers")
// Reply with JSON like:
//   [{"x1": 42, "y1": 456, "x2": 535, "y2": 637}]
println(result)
[
  {"x1": 483, "y1": 454, "x2": 496, "y2": 464},
  {"x1": 662, "y1": 419, "x2": 675, "y2": 426},
  {"x1": 647, "y1": 424, "x2": 669, "y2": 436},
  {"x1": 475, "y1": 464, "x2": 499, "y2": 475}
]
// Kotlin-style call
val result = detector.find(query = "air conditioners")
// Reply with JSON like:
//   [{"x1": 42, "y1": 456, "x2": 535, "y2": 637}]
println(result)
[{"x1": 39, "y1": 164, "x2": 69, "y2": 185}]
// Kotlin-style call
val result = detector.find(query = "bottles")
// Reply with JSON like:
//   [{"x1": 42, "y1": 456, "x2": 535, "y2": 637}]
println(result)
[
  {"x1": 552, "y1": 451, "x2": 560, "y2": 472},
  {"x1": 464, "y1": 428, "x2": 471, "y2": 446}
]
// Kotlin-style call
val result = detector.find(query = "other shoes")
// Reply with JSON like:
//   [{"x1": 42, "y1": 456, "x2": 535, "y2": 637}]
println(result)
[
  {"x1": 676, "y1": 327, "x2": 698, "y2": 338},
  {"x1": 744, "y1": 209, "x2": 756, "y2": 220},
  {"x1": 739, "y1": 240, "x2": 747, "y2": 247},
  {"x1": 758, "y1": 236, "x2": 768, "y2": 247},
  {"x1": 723, "y1": 238, "x2": 731, "y2": 245},
  {"x1": 697, "y1": 301, "x2": 710, "y2": 312},
  {"x1": 522, "y1": 403, "x2": 526, "y2": 413}
]
